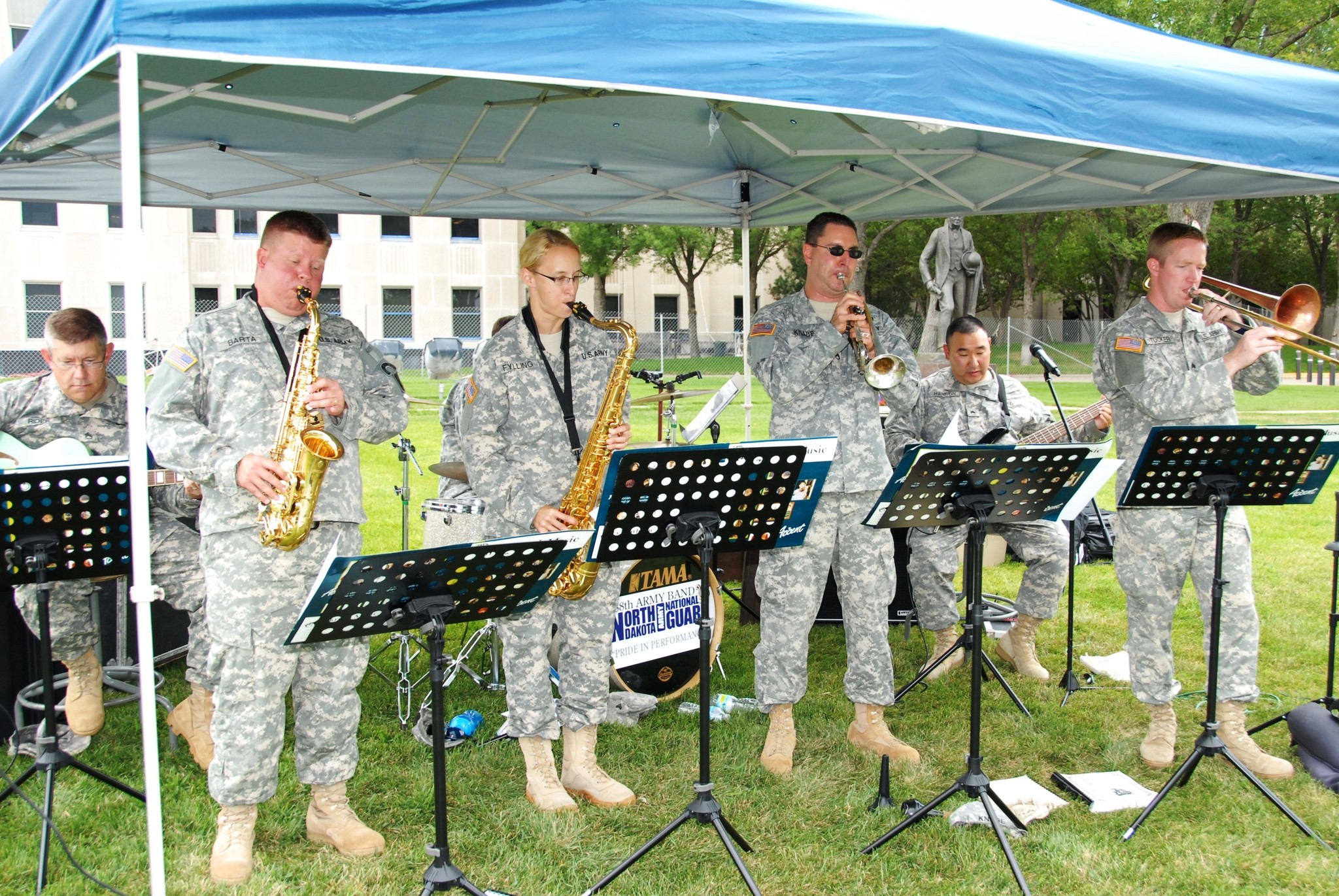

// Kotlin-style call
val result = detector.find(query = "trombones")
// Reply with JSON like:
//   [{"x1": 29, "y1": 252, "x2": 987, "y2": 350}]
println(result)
[{"x1": 1141, "y1": 268, "x2": 1339, "y2": 367}]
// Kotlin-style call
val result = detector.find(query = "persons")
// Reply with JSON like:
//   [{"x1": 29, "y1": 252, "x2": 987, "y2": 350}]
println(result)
[
  {"x1": 746, "y1": 211, "x2": 923, "y2": 778},
  {"x1": 459, "y1": 227, "x2": 638, "y2": 815},
  {"x1": 0, "y1": 306, "x2": 218, "y2": 773},
  {"x1": 882, "y1": 315, "x2": 1114, "y2": 681},
  {"x1": 1091, "y1": 222, "x2": 1295, "y2": 780},
  {"x1": 918, "y1": 215, "x2": 983, "y2": 354},
  {"x1": 143, "y1": 210, "x2": 412, "y2": 889},
  {"x1": 436, "y1": 315, "x2": 520, "y2": 500}
]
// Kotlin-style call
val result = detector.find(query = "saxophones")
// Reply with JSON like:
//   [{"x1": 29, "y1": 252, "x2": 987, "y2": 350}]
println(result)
[
  {"x1": 547, "y1": 297, "x2": 639, "y2": 600},
  {"x1": 256, "y1": 285, "x2": 344, "y2": 551}
]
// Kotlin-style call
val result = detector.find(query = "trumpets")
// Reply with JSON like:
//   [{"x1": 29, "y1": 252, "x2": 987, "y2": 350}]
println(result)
[{"x1": 836, "y1": 272, "x2": 906, "y2": 390}]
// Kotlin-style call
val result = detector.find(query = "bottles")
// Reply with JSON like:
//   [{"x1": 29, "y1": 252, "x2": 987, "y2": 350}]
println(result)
[
  {"x1": 677, "y1": 692, "x2": 761, "y2": 724},
  {"x1": 445, "y1": 708, "x2": 484, "y2": 742}
]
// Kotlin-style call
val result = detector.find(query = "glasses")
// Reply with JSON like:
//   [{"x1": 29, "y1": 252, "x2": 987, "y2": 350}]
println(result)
[
  {"x1": 808, "y1": 243, "x2": 862, "y2": 259},
  {"x1": 49, "y1": 347, "x2": 107, "y2": 373},
  {"x1": 530, "y1": 270, "x2": 589, "y2": 285}
]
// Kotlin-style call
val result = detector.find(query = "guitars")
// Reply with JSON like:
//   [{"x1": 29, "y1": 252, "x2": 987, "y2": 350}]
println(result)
[
  {"x1": 0, "y1": 426, "x2": 188, "y2": 487},
  {"x1": 938, "y1": 396, "x2": 1111, "y2": 445}
]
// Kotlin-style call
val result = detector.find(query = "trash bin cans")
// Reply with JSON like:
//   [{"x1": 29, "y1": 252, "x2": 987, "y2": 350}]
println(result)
[{"x1": 713, "y1": 342, "x2": 727, "y2": 356}]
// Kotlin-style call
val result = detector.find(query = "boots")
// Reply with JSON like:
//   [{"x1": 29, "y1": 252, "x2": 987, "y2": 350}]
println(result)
[
  {"x1": 61, "y1": 645, "x2": 106, "y2": 737},
  {"x1": 305, "y1": 781, "x2": 386, "y2": 860},
  {"x1": 518, "y1": 735, "x2": 579, "y2": 814},
  {"x1": 917, "y1": 625, "x2": 965, "y2": 685},
  {"x1": 1215, "y1": 701, "x2": 1294, "y2": 779},
  {"x1": 846, "y1": 702, "x2": 921, "y2": 765},
  {"x1": 208, "y1": 801, "x2": 258, "y2": 887},
  {"x1": 1140, "y1": 701, "x2": 1178, "y2": 767},
  {"x1": 994, "y1": 613, "x2": 1050, "y2": 681},
  {"x1": 166, "y1": 682, "x2": 217, "y2": 774},
  {"x1": 759, "y1": 702, "x2": 796, "y2": 778},
  {"x1": 561, "y1": 723, "x2": 637, "y2": 809}
]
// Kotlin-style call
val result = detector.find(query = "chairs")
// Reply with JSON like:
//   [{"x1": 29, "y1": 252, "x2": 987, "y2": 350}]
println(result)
[
  {"x1": 904, "y1": 535, "x2": 1024, "y2": 641},
  {"x1": 14, "y1": 572, "x2": 180, "y2": 753}
]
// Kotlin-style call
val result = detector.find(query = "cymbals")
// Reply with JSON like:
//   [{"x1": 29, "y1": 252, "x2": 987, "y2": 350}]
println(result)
[
  {"x1": 428, "y1": 462, "x2": 467, "y2": 482},
  {"x1": 620, "y1": 388, "x2": 714, "y2": 404}
]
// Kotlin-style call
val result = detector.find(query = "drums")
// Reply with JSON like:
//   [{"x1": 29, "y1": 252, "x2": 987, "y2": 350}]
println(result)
[
  {"x1": 422, "y1": 499, "x2": 486, "y2": 550},
  {"x1": 609, "y1": 554, "x2": 724, "y2": 704}
]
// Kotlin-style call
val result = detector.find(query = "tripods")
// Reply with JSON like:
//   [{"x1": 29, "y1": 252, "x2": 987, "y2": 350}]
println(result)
[
  {"x1": 855, "y1": 444, "x2": 1092, "y2": 896},
  {"x1": 679, "y1": 370, "x2": 761, "y2": 621},
  {"x1": 414, "y1": 618, "x2": 560, "y2": 725},
  {"x1": 1037, "y1": 372, "x2": 1133, "y2": 709},
  {"x1": 1248, "y1": 492, "x2": 1339, "y2": 733},
  {"x1": 576, "y1": 446, "x2": 809, "y2": 895},
  {"x1": 0, "y1": 465, "x2": 149, "y2": 895},
  {"x1": 367, "y1": 433, "x2": 489, "y2": 734},
  {"x1": 1117, "y1": 424, "x2": 1335, "y2": 857},
  {"x1": 895, "y1": 522, "x2": 1032, "y2": 719},
  {"x1": 305, "y1": 539, "x2": 568, "y2": 895}
]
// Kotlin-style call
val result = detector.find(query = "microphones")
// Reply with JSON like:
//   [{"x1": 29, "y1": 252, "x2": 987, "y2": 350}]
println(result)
[{"x1": 1030, "y1": 343, "x2": 1061, "y2": 377}]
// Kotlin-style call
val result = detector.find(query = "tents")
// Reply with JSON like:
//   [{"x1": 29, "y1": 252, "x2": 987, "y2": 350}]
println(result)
[{"x1": 0, "y1": 2, "x2": 1339, "y2": 895}]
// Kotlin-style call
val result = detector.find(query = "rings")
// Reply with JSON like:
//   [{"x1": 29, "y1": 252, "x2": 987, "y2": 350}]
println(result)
[
  {"x1": 333, "y1": 398, "x2": 337, "y2": 405},
  {"x1": 625, "y1": 441, "x2": 629, "y2": 445},
  {"x1": 192, "y1": 488, "x2": 198, "y2": 490},
  {"x1": 1215, "y1": 305, "x2": 1222, "y2": 311}
]
